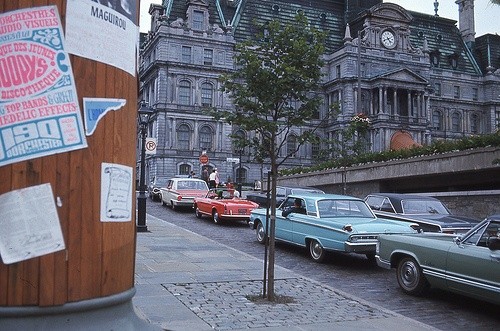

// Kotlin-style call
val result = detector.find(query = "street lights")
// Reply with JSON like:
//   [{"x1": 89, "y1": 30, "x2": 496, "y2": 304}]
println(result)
[{"x1": 137, "y1": 99, "x2": 155, "y2": 233}]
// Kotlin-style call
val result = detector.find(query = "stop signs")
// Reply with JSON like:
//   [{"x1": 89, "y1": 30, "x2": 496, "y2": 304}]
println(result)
[{"x1": 199, "y1": 154, "x2": 209, "y2": 166}]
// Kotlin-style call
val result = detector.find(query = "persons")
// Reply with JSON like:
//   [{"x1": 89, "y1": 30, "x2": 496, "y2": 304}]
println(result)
[
  {"x1": 214, "y1": 189, "x2": 223, "y2": 200},
  {"x1": 282, "y1": 198, "x2": 306, "y2": 216},
  {"x1": 201, "y1": 166, "x2": 219, "y2": 189},
  {"x1": 187, "y1": 170, "x2": 196, "y2": 178},
  {"x1": 254, "y1": 180, "x2": 262, "y2": 189}
]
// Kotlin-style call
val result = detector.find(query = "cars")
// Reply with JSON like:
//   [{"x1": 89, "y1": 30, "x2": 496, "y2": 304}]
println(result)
[
  {"x1": 374, "y1": 214, "x2": 500, "y2": 310},
  {"x1": 193, "y1": 187, "x2": 260, "y2": 226},
  {"x1": 248, "y1": 193, "x2": 422, "y2": 264},
  {"x1": 246, "y1": 185, "x2": 349, "y2": 212},
  {"x1": 148, "y1": 176, "x2": 171, "y2": 202},
  {"x1": 160, "y1": 177, "x2": 219, "y2": 212},
  {"x1": 332, "y1": 193, "x2": 500, "y2": 237}
]
